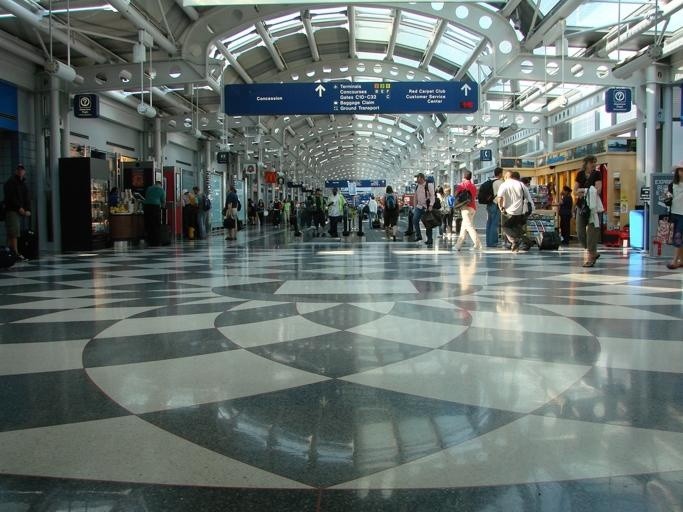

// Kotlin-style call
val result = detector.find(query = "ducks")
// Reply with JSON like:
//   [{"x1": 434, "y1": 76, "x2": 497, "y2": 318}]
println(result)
[
  {"x1": 160, "y1": 207, "x2": 171, "y2": 245},
  {"x1": 532, "y1": 214, "x2": 560, "y2": 251}
]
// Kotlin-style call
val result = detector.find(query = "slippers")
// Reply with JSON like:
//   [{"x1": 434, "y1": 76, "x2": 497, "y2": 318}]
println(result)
[
  {"x1": 386, "y1": 192, "x2": 396, "y2": 209},
  {"x1": 478, "y1": 177, "x2": 498, "y2": 204},
  {"x1": 202, "y1": 195, "x2": 210, "y2": 211}
]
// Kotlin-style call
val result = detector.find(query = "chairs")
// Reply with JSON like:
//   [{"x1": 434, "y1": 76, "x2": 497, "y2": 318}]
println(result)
[{"x1": 582, "y1": 250, "x2": 595, "y2": 267}]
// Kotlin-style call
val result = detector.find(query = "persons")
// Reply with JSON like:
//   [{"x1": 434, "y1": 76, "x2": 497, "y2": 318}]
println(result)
[
  {"x1": 191, "y1": 186, "x2": 210, "y2": 240},
  {"x1": 142, "y1": 181, "x2": 166, "y2": 247},
  {"x1": 666, "y1": 161, "x2": 683, "y2": 269},
  {"x1": 107, "y1": 186, "x2": 120, "y2": 208},
  {"x1": 547, "y1": 182, "x2": 556, "y2": 204},
  {"x1": 486, "y1": 167, "x2": 536, "y2": 251},
  {"x1": 451, "y1": 166, "x2": 482, "y2": 251},
  {"x1": 558, "y1": 186, "x2": 573, "y2": 246},
  {"x1": 3, "y1": 163, "x2": 31, "y2": 262},
  {"x1": 181, "y1": 187, "x2": 199, "y2": 239},
  {"x1": 572, "y1": 155, "x2": 603, "y2": 267},
  {"x1": 222, "y1": 163, "x2": 455, "y2": 245}
]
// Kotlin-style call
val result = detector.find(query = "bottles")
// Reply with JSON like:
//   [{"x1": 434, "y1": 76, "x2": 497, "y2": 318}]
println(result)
[
  {"x1": 670, "y1": 160, "x2": 682, "y2": 169},
  {"x1": 414, "y1": 172, "x2": 423, "y2": 178}
]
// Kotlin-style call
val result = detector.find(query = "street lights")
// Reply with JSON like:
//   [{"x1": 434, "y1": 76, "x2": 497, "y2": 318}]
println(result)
[
  {"x1": 223, "y1": 216, "x2": 235, "y2": 228},
  {"x1": 454, "y1": 189, "x2": 471, "y2": 209},
  {"x1": 579, "y1": 201, "x2": 590, "y2": 217},
  {"x1": 421, "y1": 209, "x2": 441, "y2": 229},
  {"x1": 425, "y1": 197, "x2": 440, "y2": 210},
  {"x1": 522, "y1": 199, "x2": 531, "y2": 215}
]
